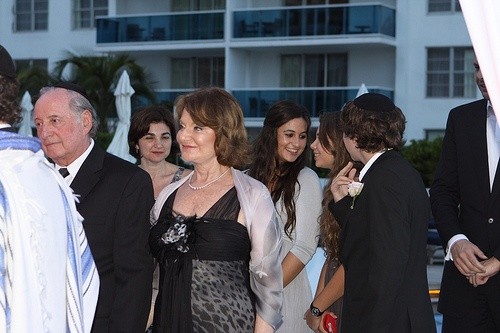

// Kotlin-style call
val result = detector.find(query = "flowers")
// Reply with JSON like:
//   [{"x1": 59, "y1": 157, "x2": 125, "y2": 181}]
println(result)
[{"x1": 348, "y1": 181, "x2": 364, "y2": 209}]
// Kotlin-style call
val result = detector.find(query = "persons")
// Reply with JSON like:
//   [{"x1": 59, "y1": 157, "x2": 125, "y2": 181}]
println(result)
[
  {"x1": 126, "y1": 102, "x2": 195, "y2": 200},
  {"x1": 0, "y1": 45, "x2": 101, "y2": 333},
  {"x1": 429, "y1": 52, "x2": 500, "y2": 333},
  {"x1": 330, "y1": 92, "x2": 439, "y2": 333},
  {"x1": 239, "y1": 100, "x2": 365, "y2": 333},
  {"x1": 33, "y1": 87, "x2": 155, "y2": 333},
  {"x1": 149, "y1": 87, "x2": 283, "y2": 333}
]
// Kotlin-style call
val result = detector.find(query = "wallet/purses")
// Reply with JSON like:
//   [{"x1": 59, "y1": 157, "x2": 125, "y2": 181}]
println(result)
[{"x1": 322, "y1": 311, "x2": 338, "y2": 333}]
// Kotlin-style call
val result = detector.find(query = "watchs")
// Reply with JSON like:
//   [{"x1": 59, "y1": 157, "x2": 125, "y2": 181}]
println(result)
[{"x1": 309, "y1": 299, "x2": 325, "y2": 317}]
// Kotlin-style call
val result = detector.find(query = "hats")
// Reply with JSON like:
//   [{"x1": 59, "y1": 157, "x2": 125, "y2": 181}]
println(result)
[{"x1": 0, "y1": 44, "x2": 15, "y2": 77}]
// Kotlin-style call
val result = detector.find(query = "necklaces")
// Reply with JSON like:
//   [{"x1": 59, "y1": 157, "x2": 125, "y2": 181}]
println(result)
[{"x1": 187, "y1": 165, "x2": 232, "y2": 191}]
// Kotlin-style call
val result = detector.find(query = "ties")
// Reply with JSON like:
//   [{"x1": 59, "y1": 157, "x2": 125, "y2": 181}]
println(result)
[{"x1": 58, "y1": 167, "x2": 70, "y2": 178}]
